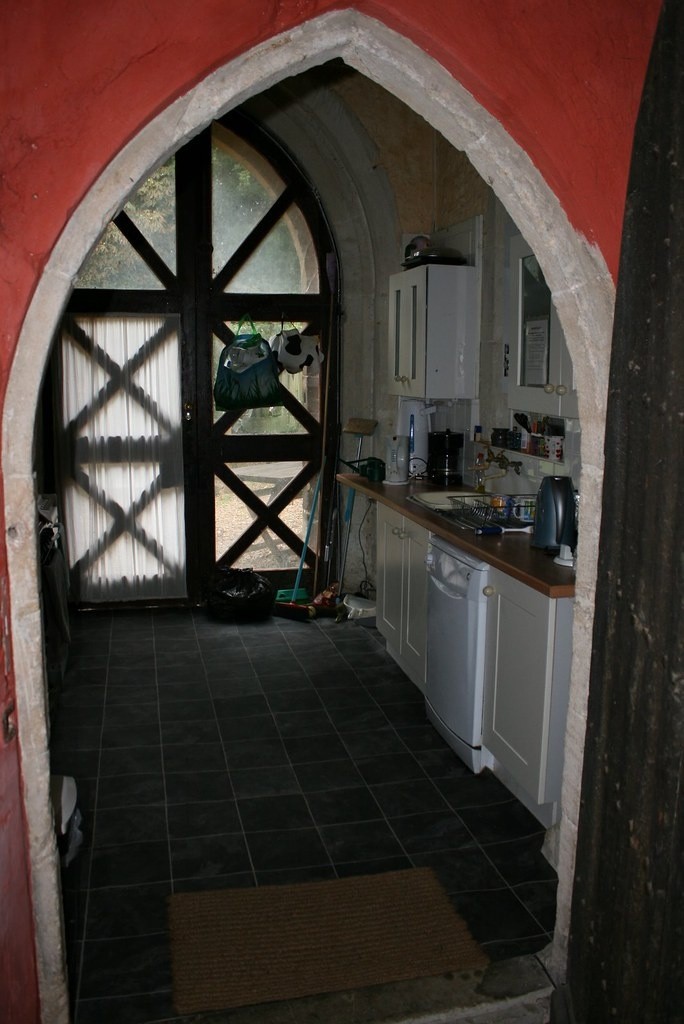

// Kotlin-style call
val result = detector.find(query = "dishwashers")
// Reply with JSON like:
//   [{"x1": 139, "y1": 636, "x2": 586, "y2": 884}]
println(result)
[{"x1": 422, "y1": 536, "x2": 489, "y2": 774}]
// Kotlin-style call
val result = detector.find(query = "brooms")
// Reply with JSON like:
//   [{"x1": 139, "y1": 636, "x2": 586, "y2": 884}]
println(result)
[
  {"x1": 334, "y1": 416, "x2": 380, "y2": 608},
  {"x1": 267, "y1": 457, "x2": 326, "y2": 621}
]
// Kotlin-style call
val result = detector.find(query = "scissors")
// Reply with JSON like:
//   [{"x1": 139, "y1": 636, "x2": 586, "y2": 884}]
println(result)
[{"x1": 513, "y1": 412, "x2": 532, "y2": 434}]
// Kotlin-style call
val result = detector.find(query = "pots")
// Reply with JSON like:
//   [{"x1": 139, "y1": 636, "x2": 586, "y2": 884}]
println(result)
[{"x1": 401, "y1": 246, "x2": 468, "y2": 270}]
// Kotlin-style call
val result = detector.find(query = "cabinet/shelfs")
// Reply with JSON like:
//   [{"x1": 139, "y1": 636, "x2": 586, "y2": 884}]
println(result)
[
  {"x1": 506, "y1": 233, "x2": 581, "y2": 418},
  {"x1": 374, "y1": 501, "x2": 434, "y2": 684},
  {"x1": 482, "y1": 563, "x2": 555, "y2": 808},
  {"x1": 386, "y1": 263, "x2": 477, "y2": 399}
]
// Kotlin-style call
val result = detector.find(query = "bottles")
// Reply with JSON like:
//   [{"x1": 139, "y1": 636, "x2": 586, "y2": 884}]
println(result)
[
  {"x1": 473, "y1": 426, "x2": 483, "y2": 442},
  {"x1": 472, "y1": 453, "x2": 486, "y2": 491}
]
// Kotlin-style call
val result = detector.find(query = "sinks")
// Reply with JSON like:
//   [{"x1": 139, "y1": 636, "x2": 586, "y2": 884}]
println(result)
[{"x1": 409, "y1": 487, "x2": 500, "y2": 515}]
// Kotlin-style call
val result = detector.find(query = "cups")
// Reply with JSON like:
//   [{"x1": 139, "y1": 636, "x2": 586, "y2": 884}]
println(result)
[
  {"x1": 505, "y1": 426, "x2": 565, "y2": 461},
  {"x1": 513, "y1": 499, "x2": 536, "y2": 523},
  {"x1": 489, "y1": 494, "x2": 513, "y2": 519}
]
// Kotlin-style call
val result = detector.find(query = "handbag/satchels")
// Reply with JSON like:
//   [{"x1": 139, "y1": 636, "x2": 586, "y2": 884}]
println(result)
[
  {"x1": 270, "y1": 316, "x2": 321, "y2": 375},
  {"x1": 213, "y1": 315, "x2": 283, "y2": 409}
]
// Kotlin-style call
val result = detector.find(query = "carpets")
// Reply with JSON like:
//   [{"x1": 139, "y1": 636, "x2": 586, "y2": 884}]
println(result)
[{"x1": 173, "y1": 864, "x2": 491, "y2": 1016}]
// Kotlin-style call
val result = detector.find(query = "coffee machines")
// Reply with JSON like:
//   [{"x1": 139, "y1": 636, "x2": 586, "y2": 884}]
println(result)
[{"x1": 426, "y1": 428, "x2": 464, "y2": 488}]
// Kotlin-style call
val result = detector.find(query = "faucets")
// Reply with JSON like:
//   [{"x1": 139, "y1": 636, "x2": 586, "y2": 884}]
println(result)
[{"x1": 475, "y1": 466, "x2": 512, "y2": 487}]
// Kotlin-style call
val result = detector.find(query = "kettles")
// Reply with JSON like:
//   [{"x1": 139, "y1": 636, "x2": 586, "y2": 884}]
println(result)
[
  {"x1": 404, "y1": 234, "x2": 433, "y2": 268},
  {"x1": 394, "y1": 399, "x2": 438, "y2": 478},
  {"x1": 529, "y1": 475, "x2": 577, "y2": 552},
  {"x1": 384, "y1": 435, "x2": 411, "y2": 484}
]
